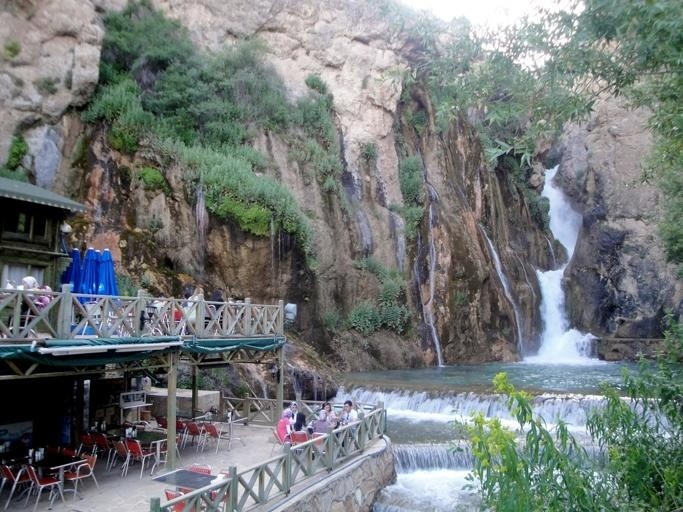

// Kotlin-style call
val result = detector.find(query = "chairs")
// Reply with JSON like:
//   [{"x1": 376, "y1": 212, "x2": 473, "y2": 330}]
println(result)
[
  {"x1": 269, "y1": 430, "x2": 328, "y2": 458},
  {"x1": 0, "y1": 413, "x2": 230, "y2": 512}
]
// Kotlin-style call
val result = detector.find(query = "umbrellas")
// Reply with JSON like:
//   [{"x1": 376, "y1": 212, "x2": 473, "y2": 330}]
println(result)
[{"x1": 63, "y1": 246, "x2": 119, "y2": 325}]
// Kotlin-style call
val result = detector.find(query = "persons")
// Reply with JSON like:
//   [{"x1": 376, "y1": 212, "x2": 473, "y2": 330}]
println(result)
[
  {"x1": 288, "y1": 401, "x2": 308, "y2": 431},
  {"x1": 339, "y1": 400, "x2": 358, "y2": 430},
  {"x1": 312, "y1": 409, "x2": 332, "y2": 433},
  {"x1": 318, "y1": 402, "x2": 336, "y2": 421},
  {"x1": 10, "y1": 431, "x2": 32, "y2": 463},
  {"x1": 138, "y1": 284, "x2": 224, "y2": 334},
  {"x1": 277, "y1": 409, "x2": 293, "y2": 442}
]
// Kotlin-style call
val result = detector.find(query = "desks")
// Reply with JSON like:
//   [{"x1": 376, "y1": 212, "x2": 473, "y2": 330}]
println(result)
[{"x1": 194, "y1": 413, "x2": 248, "y2": 454}]
